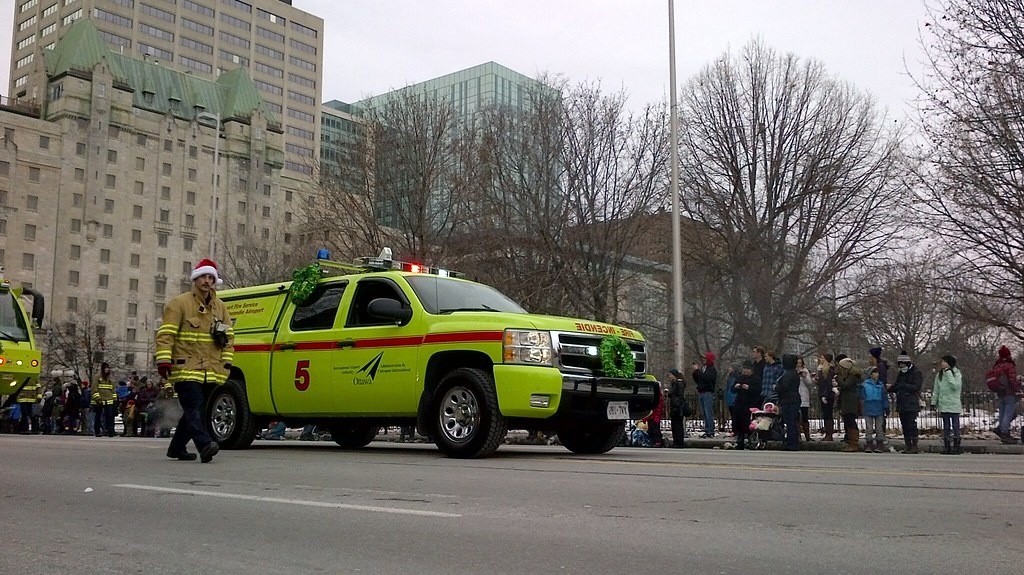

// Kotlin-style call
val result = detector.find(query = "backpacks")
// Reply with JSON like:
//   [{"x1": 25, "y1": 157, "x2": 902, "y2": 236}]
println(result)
[{"x1": 986, "y1": 366, "x2": 1008, "y2": 392}]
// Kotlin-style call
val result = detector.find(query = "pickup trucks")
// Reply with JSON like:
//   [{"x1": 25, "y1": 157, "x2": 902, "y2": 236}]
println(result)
[{"x1": 200, "y1": 245, "x2": 664, "y2": 464}]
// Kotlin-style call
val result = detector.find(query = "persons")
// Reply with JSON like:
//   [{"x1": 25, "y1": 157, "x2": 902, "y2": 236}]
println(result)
[
  {"x1": 725, "y1": 346, "x2": 812, "y2": 451},
  {"x1": 8, "y1": 362, "x2": 173, "y2": 437},
  {"x1": 814, "y1": 348, "x2": 889, "y2": 452},
  {"x1": 668, "y1": 369, "x2": 686, "y2": 448},
  {"x1": 642, "y1": 383, "x2": 665, "y2": 448},
  {"x1": 260, "y1": 424, "x2": 320, "y2": 440},
  {"x1": 993, "y1": 346, "x2": 1019, "y2": 443},
  {"x1": 399, "y1": 426, "x2": 415, "y2": 442},
  {"x1": 156, "y1": 258, "x2": 235, "y2": 462},
  {"x1": 692, "y1": 352, "x2": 717, "y2": 437},
  {"x1": 887, "y1": 351, "x2": 923, "y2": 453},
  {"x1": 931, "y1": 356, "x2": 962, "y2": 454}
]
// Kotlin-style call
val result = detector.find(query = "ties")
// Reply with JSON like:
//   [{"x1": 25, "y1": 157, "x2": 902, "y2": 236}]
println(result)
[{"x1": 942, "y1": 355, "x2": 956, "y2": 368}]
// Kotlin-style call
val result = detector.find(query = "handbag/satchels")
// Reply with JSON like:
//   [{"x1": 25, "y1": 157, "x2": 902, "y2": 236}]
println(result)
[
  {"x1": 682, "y1": 400, "x2": 693, "y2": 417},
  {"x1": 632, "y1": 430, "x2": 652, "y2": 447}
]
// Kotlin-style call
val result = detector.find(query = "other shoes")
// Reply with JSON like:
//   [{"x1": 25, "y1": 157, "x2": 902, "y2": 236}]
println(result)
[
  {"x1": 200, "y1": 441, "x2": 219, "y2": 463},
  {"x1": 653, "y1": 433, "x2": 713, "y2": 449},
  {"x1": 167, "y1": 447, "x2": 197, "y2": 459}
]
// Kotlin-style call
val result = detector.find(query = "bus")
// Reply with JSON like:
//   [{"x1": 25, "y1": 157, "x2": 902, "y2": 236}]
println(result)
[{"x1": 0, "y1": 277, "x2": 46, "y2": 434}]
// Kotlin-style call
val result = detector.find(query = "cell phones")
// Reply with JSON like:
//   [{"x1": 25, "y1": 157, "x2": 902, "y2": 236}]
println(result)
[{"x1": 692, "y1": 362, "x2": 695, "y2": 365}]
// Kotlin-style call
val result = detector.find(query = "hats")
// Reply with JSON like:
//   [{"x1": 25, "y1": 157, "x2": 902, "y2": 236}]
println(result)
[
  {"x1": 670, "y1": 369, "x2": 678, "y2": 376},
  {"x1": 119, "y1": 381, "x2": 127, "y2": 386},
  {"x1": 700, "y1": 352, "x2": 715, "y2": 361},
  {"x1": 101, "y1": 362, "x2": 110, "y2": 372},
  {"x1": 165, "y1": 383, "x2": 172, "y2": 389},
  {"x1": 192, "y1": 259, "x2": 223, "y2": 287},
  {"x1": 998, "y1": 345, "x2": 1011, "y2": 358},
  {"x1": 839, "y1": 358, "x2": 852, "y2": 369},
  {"x1": 867, "y1": 366, "x2": 877, "y2": 375},
  {"x1": 835, "y1": 354, "x2": 847, "y2": 362},
  {"x1": 742, "y1": 360, "x2": 752, "y2": 369},
  {"x1": 128, "y1": 400, "x2": 135, "y2": 405},
  {"x1": 82, "y1": 381, "x2": 89, "y2": 386},
  {"x1": 870, "y1": 347, "x2": 881, "y2": 358},
  {"x1": 822, "y1": 353, "x2": 833, "y2": 361},
  {"x1": 897, "y1": 350, "x2": 912, "y2": 367}
]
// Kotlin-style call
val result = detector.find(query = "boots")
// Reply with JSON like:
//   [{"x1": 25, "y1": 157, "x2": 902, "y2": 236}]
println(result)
[{"x1": 795, "y1": 420, "x2": 1020, "y2": 454}]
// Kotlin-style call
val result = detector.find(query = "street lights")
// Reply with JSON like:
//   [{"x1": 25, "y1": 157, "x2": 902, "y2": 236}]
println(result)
[{"x1": 200, "y1": 108, "x2": 220, "y2": 257}]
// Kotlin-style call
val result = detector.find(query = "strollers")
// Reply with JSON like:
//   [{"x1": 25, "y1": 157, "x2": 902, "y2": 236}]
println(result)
[{"x1": 747, "y1": 389, "x2": 789, "y2": 450}]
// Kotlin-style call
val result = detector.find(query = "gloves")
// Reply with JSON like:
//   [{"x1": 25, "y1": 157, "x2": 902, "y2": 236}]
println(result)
[{"x1": 158, "y1": 363, "x2": 171, "y2": 380}]
